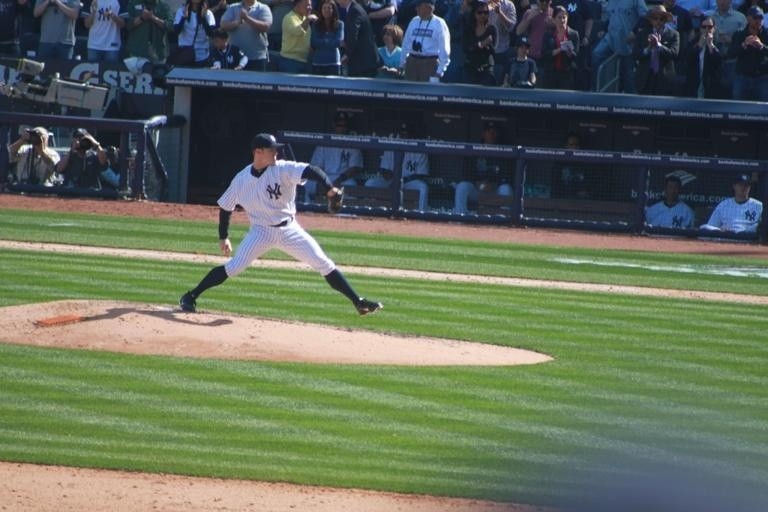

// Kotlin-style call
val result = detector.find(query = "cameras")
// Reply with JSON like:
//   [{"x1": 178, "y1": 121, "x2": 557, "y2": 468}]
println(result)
[
  {"x1": 26, "y1": 129, "x2": 41, "y2": 144},
  {"x1": 80, "y1": 136, "x2": 92, "y2": 151}
]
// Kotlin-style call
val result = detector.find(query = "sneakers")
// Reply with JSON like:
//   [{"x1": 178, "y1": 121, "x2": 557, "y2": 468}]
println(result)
[
  {"x1": 180, "y1": 291, "x2": 197, "y2": 313},
  {"x1": 357, "y1": 298, "x2": 384, "y2": 317}
]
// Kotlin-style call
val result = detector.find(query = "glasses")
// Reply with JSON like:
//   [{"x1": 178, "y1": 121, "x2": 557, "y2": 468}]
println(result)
[
  {"x1": 700, "y1": 24, "x2": 714, "y2": 30},
  {"x1": 477, "y1": 10, "x2": 489, "y2": 15}
]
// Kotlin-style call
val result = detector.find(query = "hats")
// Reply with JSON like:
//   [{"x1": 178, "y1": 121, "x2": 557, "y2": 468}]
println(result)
[
  {"x1": 733, "y1": 173, "x2": 751, "y2": 185},
  {"x1": 251, "y1": 133, "x2": 286, "y2": 150},
  {"x1": 410, "y1": 0, "x2": 436, "y2": 6},
  {"x1": 208, "y1": 28, "x2": 228, "y2": 38},
  {"x1": 334, "y1": 112, "x2": 348, "y2": 122},
  {"x1": 647, "y1": 5, "x2": 673, "y2": 23},
  {"x1": 747, "y1": 5, "x2": 764, "y2": 17},
  {"x1": 515, "y1": 37, "x2": 530, "y2": 46}
]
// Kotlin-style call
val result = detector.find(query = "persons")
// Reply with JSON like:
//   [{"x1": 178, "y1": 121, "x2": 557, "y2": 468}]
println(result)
[
  {"x1": 550, "y1": 134, "x2": 592, "y2": 199},
  {"x1": 644, "y1": 176, "x2": 695, "y2": 230},
  {"x1": 0, "y1": 1, "x2": 768, "y2": 101},
  {"x1": 7, "y1": 126, "x2": 61, "y2": 187},
  {"x1": 304, "y1": 112, "x2": 364, "y2": 203},
  {"x1": 55, "y1": 128, "x2": 108, "y2": 191},
  {"x1": 699, "y1": 172, "x2": 764, "y2": 234},
  {"x1": 179, "y1": 132, "x2": 383, "y2": 317},
  {"x1": 364, "y1": 123, "x2": 429, "y2": 213},
  {"x1": 454, "y1": 129, "x2": 514, "y2": 217}
]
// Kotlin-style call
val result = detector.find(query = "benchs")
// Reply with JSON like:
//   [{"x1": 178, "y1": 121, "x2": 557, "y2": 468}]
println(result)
[
  {"x1": 315, "y1": 185, "x2": 419, "y2": 210},
  {"x1": 476, "y1": 195, "x2": 635, "y2": 221},
  {"x1": 692, "y1": 206, "x2": 716, "y2": 226}
]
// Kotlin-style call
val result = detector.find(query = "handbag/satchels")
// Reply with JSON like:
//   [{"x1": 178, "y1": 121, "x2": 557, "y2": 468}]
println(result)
[{"x1": 171, "y1": 46, "x2": 195, "y2": 65}]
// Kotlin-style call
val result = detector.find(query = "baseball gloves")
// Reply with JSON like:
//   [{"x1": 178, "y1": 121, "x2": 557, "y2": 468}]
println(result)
[{"x1": 326, "y1": 187, "x2": 345, "y2": 215}]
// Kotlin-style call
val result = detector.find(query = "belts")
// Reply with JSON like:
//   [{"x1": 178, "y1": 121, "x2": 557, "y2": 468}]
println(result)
[
  {"x1": 270, "y1": 216, "x2": 294, "y2": 227},
  {"x1": 410, "y1": 53, "x2": 438, "y2": 59}
]
